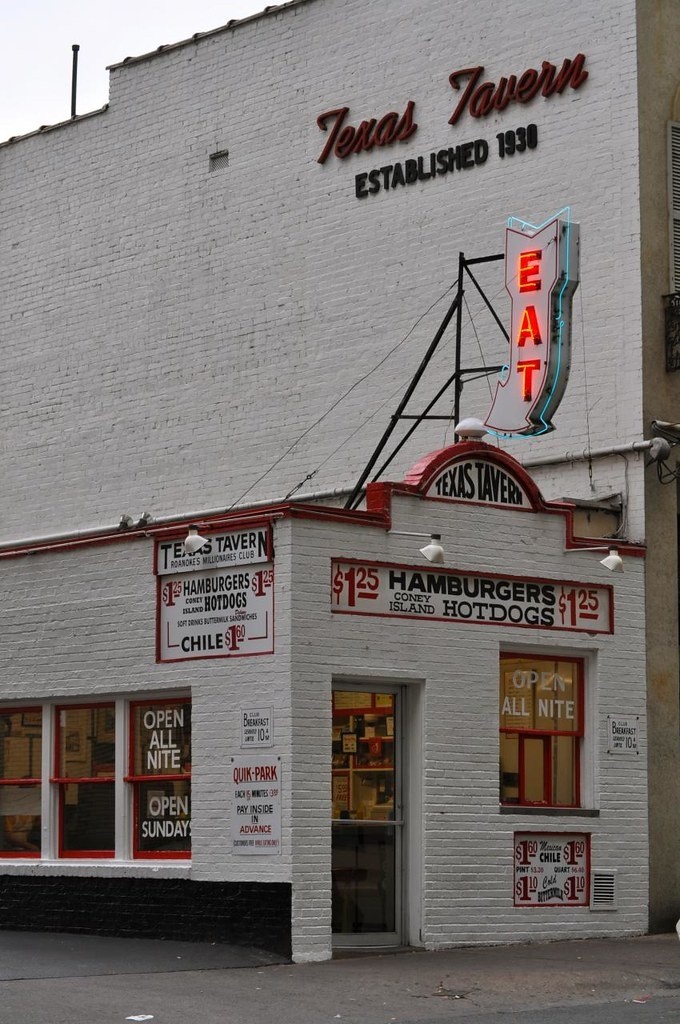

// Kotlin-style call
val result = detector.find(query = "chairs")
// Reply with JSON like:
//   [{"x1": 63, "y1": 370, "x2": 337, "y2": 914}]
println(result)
[{"x1": 330, "y1": 841, "x2": 387, "y2": 928}]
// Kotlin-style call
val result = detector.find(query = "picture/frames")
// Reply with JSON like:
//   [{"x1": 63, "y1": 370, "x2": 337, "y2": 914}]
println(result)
[
  {"x1": 21, "y1": 713, "x2": 42, "y2": 727},
  {"x1": 65, "y1": 725, "x2": 87, "y2": 762}
]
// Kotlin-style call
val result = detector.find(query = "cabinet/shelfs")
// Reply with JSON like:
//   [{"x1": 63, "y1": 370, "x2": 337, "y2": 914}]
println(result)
[{"x1": 332, "y1": 706, "x2": 395, "y2": 820}]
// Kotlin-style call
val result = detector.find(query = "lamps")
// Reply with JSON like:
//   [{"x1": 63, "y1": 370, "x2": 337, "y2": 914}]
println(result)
[
  {"x1": 136, "y1": 511, "x2": 153, "y2": 529},
  {"x1": 117, "y1": 515, "x2": 134, "y2": 531},
  {"x1": 564, "y1": 547, "x2": 623, "y2": 573},
  {"x1": 184, "y1": 522, "x2": 208, "y2": 555},
  {"x1": 385, "y1": 531, "x2": 445, "y2": 564}
]
